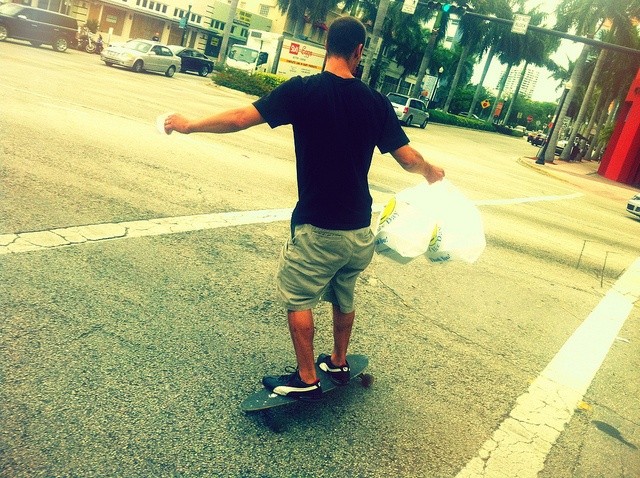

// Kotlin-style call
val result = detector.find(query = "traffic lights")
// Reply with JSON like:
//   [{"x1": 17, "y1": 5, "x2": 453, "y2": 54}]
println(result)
[{"x1": 420, "y1": 0, "x2": 470, "y2": 16}]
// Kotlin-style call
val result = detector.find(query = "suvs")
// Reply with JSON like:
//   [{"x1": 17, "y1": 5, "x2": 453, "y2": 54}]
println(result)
[
  {"x1": 459, "y1": 111, "x2": 479, "y2": 121},
  {"x1": 385, "y1": 91, "x2": 429, "y2": 129},
  {"x1": 0, "y1": 2, "x2": 79, "y2": 52}
]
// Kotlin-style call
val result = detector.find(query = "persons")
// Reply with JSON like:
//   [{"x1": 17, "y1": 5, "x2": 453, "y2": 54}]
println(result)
[{"x1": 163, "y1": 16, "x2": 442, "y2": 403}]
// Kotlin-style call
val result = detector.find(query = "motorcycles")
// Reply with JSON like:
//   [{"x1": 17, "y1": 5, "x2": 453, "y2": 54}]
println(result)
[{"x1": 86, "y1": 26, "x2": 97, "y2": 55}]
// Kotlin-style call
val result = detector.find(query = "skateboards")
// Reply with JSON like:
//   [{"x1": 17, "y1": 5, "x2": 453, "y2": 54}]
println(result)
[{"x1": 239, "y1": 355, "x2": 375, "y2": 434}]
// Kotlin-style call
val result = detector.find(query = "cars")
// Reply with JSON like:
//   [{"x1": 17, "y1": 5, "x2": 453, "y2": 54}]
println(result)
[
  {"x1": 102, "y1": 41, "x2": 180, "y2": 78},
  {"x1": 528, "y1": 130, "x2": 577, "y2": 154},
  {"x1": 625, "y1": 193, "x2": 640, "y2": 222},
  {"x1": 165, "y1": 46, "x2": 213, "y2": 77}
]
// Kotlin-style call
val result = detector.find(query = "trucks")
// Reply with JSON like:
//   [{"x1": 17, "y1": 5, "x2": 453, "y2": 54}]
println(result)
[{"x1": 221, "y1": 29, "x2": 286, "y2": 75}]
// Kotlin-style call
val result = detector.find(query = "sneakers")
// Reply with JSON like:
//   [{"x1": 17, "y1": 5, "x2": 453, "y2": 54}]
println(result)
[
  {"x1": 262, "y1": 366, "x2": 323, "y2": 402},
  {"x1": 317, "y1": 354, "x2": 351, "y2": 386}
]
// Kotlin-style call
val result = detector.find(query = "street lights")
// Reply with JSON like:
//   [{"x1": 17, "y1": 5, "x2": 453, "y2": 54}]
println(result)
[
  {"x1": 535, "y1": 80, "x2": 575, "y2": 163},
  {"x1": 178, "y1": 3, "x2": 192, "y2": 46},
  {"x1": 495, "y1": 96, "x2": 509, "y2": 126},
  {"x1": 424, "y1": 66, "x2": 446, "y2": 111}
]
